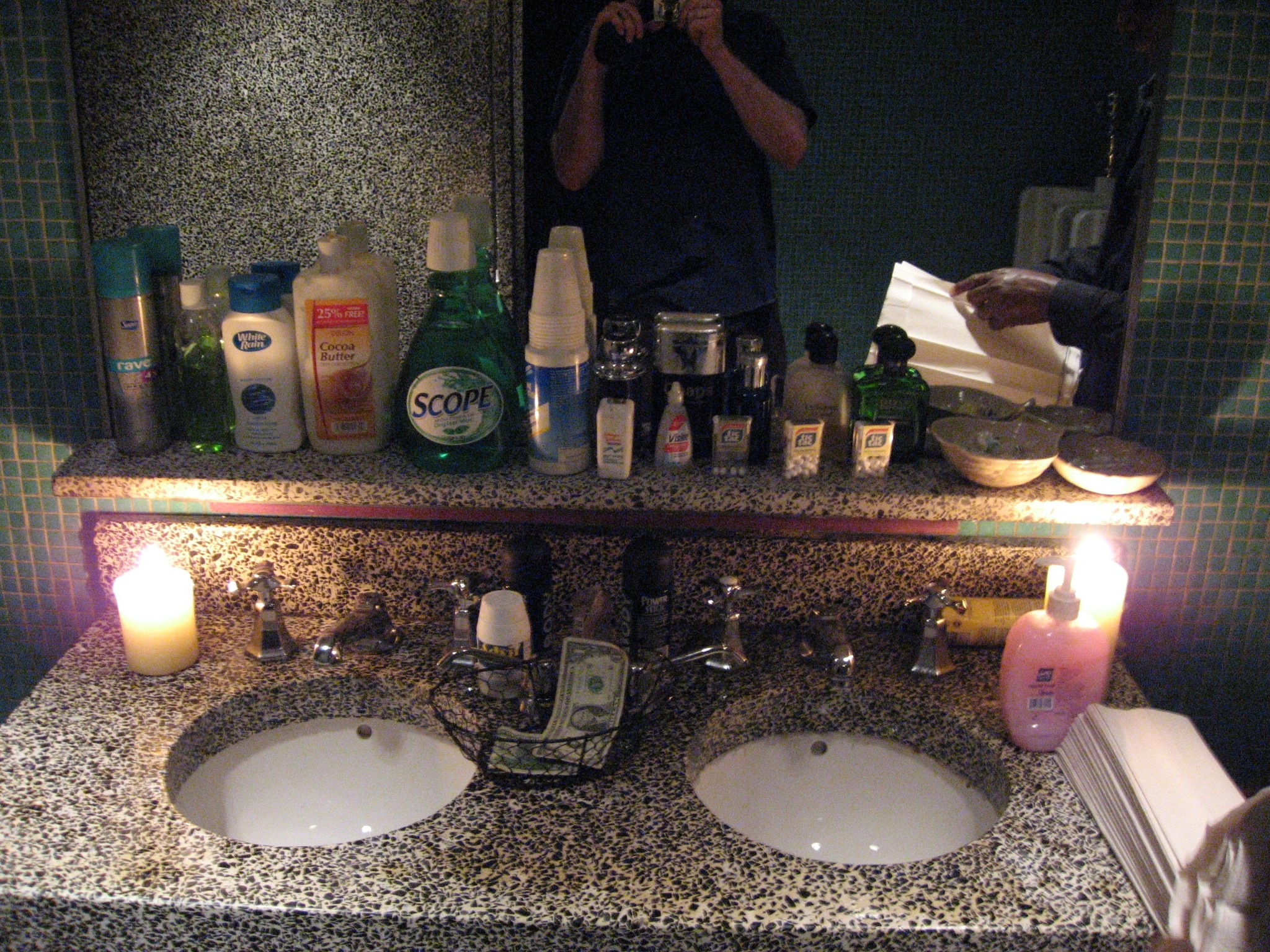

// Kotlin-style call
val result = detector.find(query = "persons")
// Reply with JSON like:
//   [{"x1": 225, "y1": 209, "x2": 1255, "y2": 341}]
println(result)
[
  {"x1": 541, "y1": 0, "x2": 814, "y2": 395},
  {"x1": 946, "y1": 0, "x2": 1173, "y2": 422}
]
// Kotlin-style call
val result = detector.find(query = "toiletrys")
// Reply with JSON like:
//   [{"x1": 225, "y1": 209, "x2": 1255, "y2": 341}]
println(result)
[
  {"x1": 595, "y1": 398, "x2": 639, "y2": 480},
  {"x1": 130, "y1": 216, "x2": 388, "y2": 441},
  {"x1": 455, "y1": 202, "x2": 518, "y2": 368},
  {"x1": 596, "y1": 312, "x2": 908, "y2": 377},
  {"x1": 590, "y1": 311, "x2": 931, "y2": 459},
  {"x1": 1004, "y1": 558, "x2": 1112, "y2": 755},
  {"x1": 87, "y1": 231, "x2": 390, "y2": 457},
  {"x1": 393, "y1": 211, "x2": 522, "y2": 477},
  {"x1": 656, "y1": 380, "x2": 696, "y2": 468}
]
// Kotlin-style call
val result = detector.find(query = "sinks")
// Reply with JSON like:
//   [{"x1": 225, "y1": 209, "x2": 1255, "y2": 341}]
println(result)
[
  {"x1": 683, "y1": 674, "x2": 1016, "y2": 867},
  {"x1": 163, "y1": 670, "x2": 483, "y2": 850}
]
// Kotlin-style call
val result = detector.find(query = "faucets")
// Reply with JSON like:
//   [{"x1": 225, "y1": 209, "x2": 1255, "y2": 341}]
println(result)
[
  {"x1": 799, "y1": 607, "x2": 858, "y2": 682},
  {"x1": 310, "y1": 592, "x2": 402, "y2": 666}
]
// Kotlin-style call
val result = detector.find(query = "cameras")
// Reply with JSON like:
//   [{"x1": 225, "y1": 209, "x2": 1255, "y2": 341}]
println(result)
[{"x1": 637, "y1": 0, "x2": 683, "y2": 23}]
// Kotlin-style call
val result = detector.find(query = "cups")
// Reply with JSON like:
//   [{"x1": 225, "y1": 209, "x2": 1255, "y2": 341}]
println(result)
[
  {"x1": 527, "y1": 248, "x2": 587, "y2": 354},
  {"x1": 1045, "y1": 556, "x2": 1129, "y2": 670},
  {"x1": 548, "y1": 226, "x2": 594, "y2": 318},
  {"x1": 523, "y1": 343, "x2": 594, "y2": 478}
]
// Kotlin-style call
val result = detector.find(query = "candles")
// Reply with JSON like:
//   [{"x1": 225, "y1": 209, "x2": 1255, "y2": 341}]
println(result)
[
  {"x1": 1043, "y1": 531, "x2": 1130, "y2": 660},
  {"x1": 111, "y1": 545, "x2": 199, "y2": 676}
]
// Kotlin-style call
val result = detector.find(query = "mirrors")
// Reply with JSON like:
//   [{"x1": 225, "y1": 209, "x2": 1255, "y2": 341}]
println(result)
[{"x1": 492, "y1": 0, "x2": 1172, "y2": 435}]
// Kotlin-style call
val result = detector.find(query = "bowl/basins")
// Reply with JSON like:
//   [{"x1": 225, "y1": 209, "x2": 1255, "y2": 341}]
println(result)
[
  {"x1": 929, "y1": 416, "x2": 1060, "y2": 488},
  {"x1": 926, "y1": 385, "x2": 1021, "y2": 423},
  {"x1": 1052, "y1": 435, "x2": 1167, "y2": 496},
  {"x1": 1021, "y1": 405, "x2": 1126, "y2": 440}
]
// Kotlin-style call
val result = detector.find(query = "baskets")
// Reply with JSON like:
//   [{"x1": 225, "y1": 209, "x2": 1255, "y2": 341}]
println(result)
[{"x1": 429, "y1": 645, "x2": 676, "y2": 788}]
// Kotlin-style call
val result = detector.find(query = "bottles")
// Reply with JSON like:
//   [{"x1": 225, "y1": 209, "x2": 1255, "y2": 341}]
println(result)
[
  {"x1": 786, "y1": 330, "x2": 849, "y2": 464},
  {"x1": 729, "y1": 333, "x2": 771, "y2": 392},
  {"x1": 850, "y1": 324, "x2": 910, "y2": 384},
  {"x1": 394, "y1": 196, "x2": 527, "y2": 474},
  {"x1": 849, "y1": 338, "x2": 931, "y2": 465},
  {"x1": 727, "y1": 349, "x2": 773, "y2": 466},
  {"x1": 596, "y1": 383, "x2": 637, "y2": 481},
  {"x1": 784, "y1": 323, "x2": 844, "y2": 379},
  {"x1": 653, "y1": 379, "x2": 692, "y2": 474},
  {"x1": 93, "y1": 220, "x2": 403, "y2": 455},
  {"x1": 475, "y1": 590, "x2": 533, "y2": 701}
]
